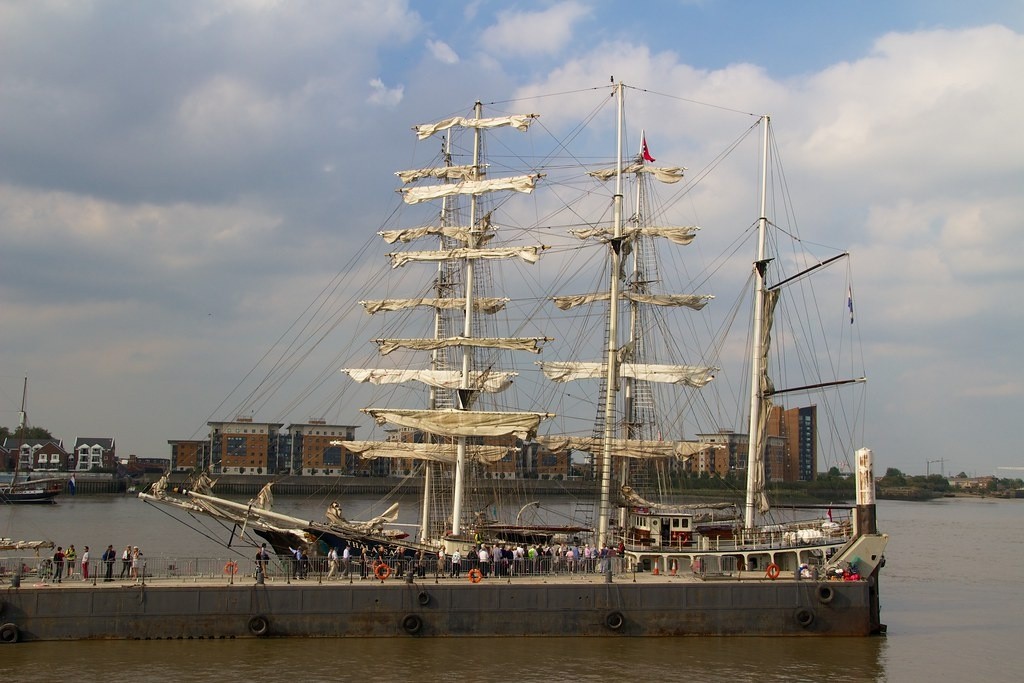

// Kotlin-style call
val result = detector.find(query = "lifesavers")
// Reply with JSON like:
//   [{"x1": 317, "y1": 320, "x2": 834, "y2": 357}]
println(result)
[
  {"x1": 604, "y1": 610, "x2": 624, "y2": 632},
  {"x1": 247, "y1": 615, "x2": 269, "y2": 636},
  {"x1": 375, "y1": 564, "x2": 391, "y2": 579},
  {"x1": 399, "y1": 614, "x2": 423, "y2": 634},
  {"x1": 224, "y1": 562, "x2": 238, "y2": 575},
  {"x1": 0, "y1": 623, "x2": 18, "y2": 643},
  {"x1": 468, "y1": 569, "x2": 482, "y2": 583},
  {"x1": 815, "y1": 584, "x2": 834, "y2": 603},
  {"x1": 767, "y1": 564, "x2": 780, "y2": 578},
  {"x1": 678, "y1": 532, "x2": 688, "y2": 545},
  {"x1": 793, "y1": 607, "x2": 814, "y2": 626},
  {"x1": 416, "y1": 590, "x2": 429, "y2": 604}
]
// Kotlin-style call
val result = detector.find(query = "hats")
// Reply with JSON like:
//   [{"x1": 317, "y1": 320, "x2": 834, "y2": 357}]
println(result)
[{"x1": 133, "y1": 545, "x2": 139, "y2": 549}]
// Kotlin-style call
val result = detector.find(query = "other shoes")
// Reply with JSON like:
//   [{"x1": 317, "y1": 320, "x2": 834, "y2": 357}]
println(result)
[{"x1": 104, "y1": 578, "x2": 115, "y2": 582}]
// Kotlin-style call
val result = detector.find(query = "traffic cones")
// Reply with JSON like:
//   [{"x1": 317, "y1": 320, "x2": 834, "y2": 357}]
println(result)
[
  {"x1": 651, "y1": 558, "x2": 663, "y2": 576},
  {"x1": 668, "y1": 557, "x2": 680, "y2": 576}
]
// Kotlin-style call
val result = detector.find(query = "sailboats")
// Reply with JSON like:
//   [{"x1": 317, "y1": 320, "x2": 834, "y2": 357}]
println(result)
[
  {"x1": 0, "y1": 371, "x2": 68, "y2": 506},
  {"x1": 129, "y1": 74, "x2": 863, "y2": 578}
]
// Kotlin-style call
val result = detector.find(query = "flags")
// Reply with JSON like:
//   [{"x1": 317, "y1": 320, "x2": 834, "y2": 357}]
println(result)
[
  {"x1": 642, "y1": 137, "x2": 655, "y2": 162},
  {"x1": 848, "y1": 286, "x2": 853, "y2": 325},
  {"x1": 826, "y1": 507, "x2": 832, "y2": 522},
  {"x1": 67, "y1": 473, "x2": 76, "y2": 495}
]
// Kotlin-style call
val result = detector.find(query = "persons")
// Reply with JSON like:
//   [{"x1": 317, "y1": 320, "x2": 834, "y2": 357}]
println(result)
[
  {"x1": 289, "y1": 546, "x2": 310, "y2": 580},
  {"x1": 450, "y1": 548, "x2": 461, "y2": 578},
  {"x1": 437, "y1": 545, "x2": 447, "y2": 578},
  {"x1": 81, "y1": 545, "x2": 90, "y2": 581},
  {"x1": 466, "y1": 539, "x2": 625, "y2": 578},
  {"x1": 341, "y1": 545, "x2": 353, "y2": 579},
  {"x1": 120, "y1": 545, "x2": 140, "y2": 580},
  {"x1": 65, "y1": 544, "x2": 76, "y2": 579},
  {"x1": 359, "y1": 545, "x2": 404, "y2": 579},
  {"x1": 325, "y1": 546, "x2": 340, "y2": 581},
  {"x1": 411, "y1": 549, "x2": 426, "y2": 579},
  {"x1": 53, "y1": 547, "x2": 65, "y2": 583},
  {"x1": 104, "y1": 544, "x2": 115, "y2": 582},
  {"x1": 252, "y1": 542, "x2": 268, "y2": 578}
]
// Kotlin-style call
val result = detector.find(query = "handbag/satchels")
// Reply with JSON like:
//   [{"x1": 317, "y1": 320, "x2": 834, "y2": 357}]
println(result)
[{"x1": 107, "y1": 558, "x2": 115, "y2": 563}]
